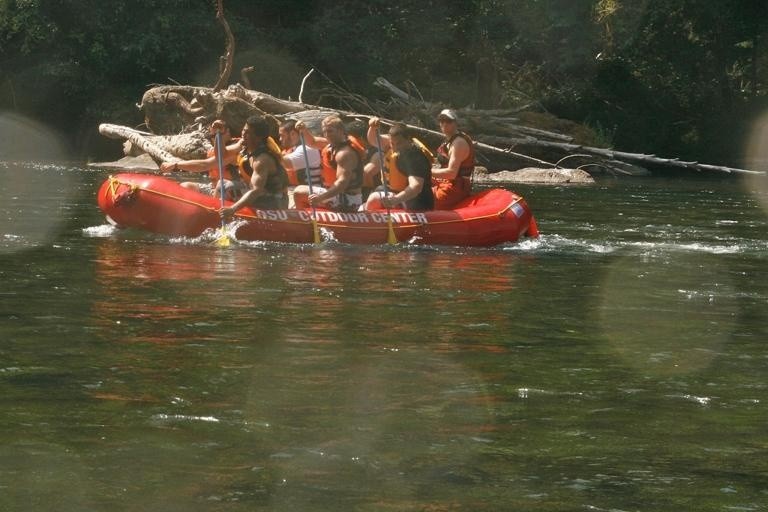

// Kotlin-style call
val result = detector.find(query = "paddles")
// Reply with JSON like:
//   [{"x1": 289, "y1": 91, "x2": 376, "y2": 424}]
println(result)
[
  {"x1": 215, "y1": 123, "x2": 231, "y2": 247},
  {"x1": 298, "y1": 123, "x2": 321, "y2": 245},
  {"x1": 89, "y1": 163, "x2": 186, "y2": 174},
  {"x1": 375, "y1": 124, "x2": 398, "y2": 246}
]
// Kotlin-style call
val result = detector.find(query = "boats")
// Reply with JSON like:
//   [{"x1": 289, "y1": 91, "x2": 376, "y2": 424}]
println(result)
[{"x1": 97, "y1": 174, "x2": 538, "y2": 245}]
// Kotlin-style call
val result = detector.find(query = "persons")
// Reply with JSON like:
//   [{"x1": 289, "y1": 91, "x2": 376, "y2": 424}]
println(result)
[
  {"x1": 211, "y1": 116, "x2": 290, "y2": 221},
  {"x1": 264, "y1": 115, "x2": 323, "y2": 186},
  {"x1": 293, "y1": 115, "x2": 382, "y2": 212},
  {"x1": 430, "y1": 109, "x2": 475, "y2": 212},
  {"x1": 366, "y1": 116, "x2": 433, "y2": 212},
  {"x1": 160, "y1": 120, "x2": 245, "y2": 197}
]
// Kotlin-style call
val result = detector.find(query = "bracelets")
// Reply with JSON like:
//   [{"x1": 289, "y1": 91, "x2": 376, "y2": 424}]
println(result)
[{"x1": 175, "y1": 162, "x2": 178, "y2": 170}]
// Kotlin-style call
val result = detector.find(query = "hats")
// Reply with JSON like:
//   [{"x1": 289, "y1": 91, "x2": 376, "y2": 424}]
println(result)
[{"x1": 438, "y1": 108, "x2": 457, "y2": 122}]
